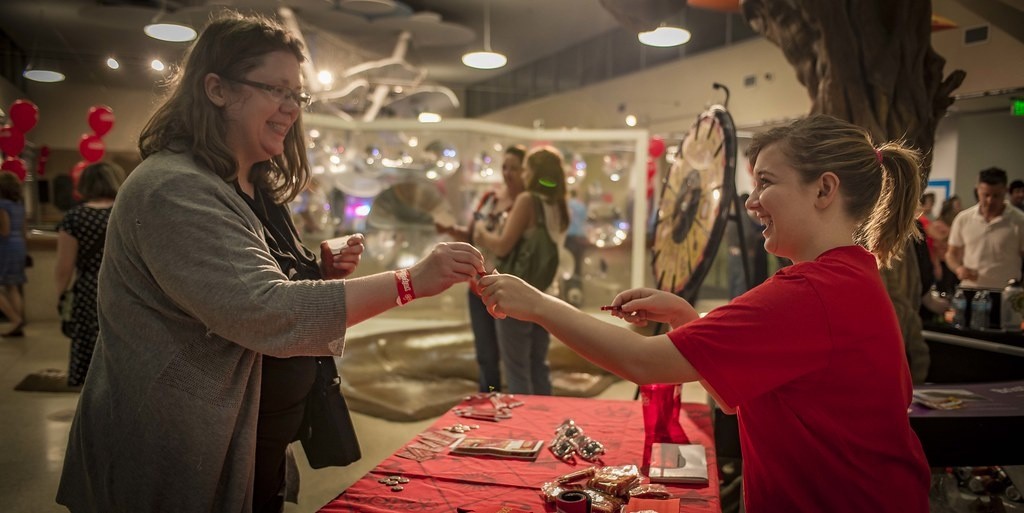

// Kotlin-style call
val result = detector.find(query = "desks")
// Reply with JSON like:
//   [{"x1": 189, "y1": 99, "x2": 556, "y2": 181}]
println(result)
[
  {"x1": 318, "y1": 395, "x2": 723, "y2": 513},
  {"x1": 915, "y1": 327, "x2": 1024, "y2": 370}
]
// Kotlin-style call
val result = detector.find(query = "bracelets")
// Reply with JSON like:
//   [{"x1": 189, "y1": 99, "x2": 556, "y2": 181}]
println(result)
[{"x1": 395, "y1": 268, "x2": 416, "y2": 306}]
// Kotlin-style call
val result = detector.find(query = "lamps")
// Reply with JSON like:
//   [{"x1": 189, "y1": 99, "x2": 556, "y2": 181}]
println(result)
[
  {"x1": 461, "y1": 0, "x2": 508, "y2": 70},
  {"x1": 143, "y1": 0, "x2": 197, "y2": 43},
  {"x1": 638, "y1": 11, "x2": 690, "y2": 47},
  {"x1": 23, "y1": 51, "x2": 65, "y2": 82}
]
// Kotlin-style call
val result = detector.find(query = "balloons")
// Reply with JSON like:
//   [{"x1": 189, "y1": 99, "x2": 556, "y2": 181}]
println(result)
[
  {"x1": 0, "y1": 124, "x2": 25, "y2": 156},
  {"x1": 89, "y1": 104, "x2": 114, "y2": 136},
  {"x1": 80, "y1": 134, "x2": 105, "y2": 162},
  {"x1": 71, "y1": 160, "x2": 93, "y2": 202},
  {"x1": 9, "y1": 99, "x2": 40, "y2": 133},
  {"x1": 37, "y1": 146, "x2": 50, "y2": 176},
  {"x1": 1, "y1": 157, "x2": 26, "y2": 181}
]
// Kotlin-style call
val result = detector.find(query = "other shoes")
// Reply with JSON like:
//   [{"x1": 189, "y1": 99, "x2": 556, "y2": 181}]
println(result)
[{"x1": 0, "y1": 320, "x2": 25, "y2": 337}]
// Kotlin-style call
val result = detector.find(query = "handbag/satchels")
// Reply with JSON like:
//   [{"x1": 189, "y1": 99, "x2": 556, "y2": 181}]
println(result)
[
  {"x1": 495, "y1": 192, "x2": 559, "y2": 291},
  {"x1": 299, "y1": 377, "x2": 361, "y2": 469}
]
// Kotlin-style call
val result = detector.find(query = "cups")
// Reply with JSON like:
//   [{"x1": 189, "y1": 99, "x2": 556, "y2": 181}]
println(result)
[
  {"x1": 639, "y1": 382, "x2": 681, "y2": 439},
  {"x1": 326, "y1": 235, "x2": 349, "y2": 258}
]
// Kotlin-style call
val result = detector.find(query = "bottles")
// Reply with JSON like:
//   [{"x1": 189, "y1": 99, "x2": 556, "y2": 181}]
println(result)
[{"x1": 951, "y1": 278, "x2": 1024, "y2": 331}]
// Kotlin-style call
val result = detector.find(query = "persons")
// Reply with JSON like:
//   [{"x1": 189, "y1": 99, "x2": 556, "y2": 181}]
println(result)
[
  {"x1": 724, "y1": 193, "x2": 768, "y2": 300},
  {"x1": 563, "y1": 190, "x2": 589, "y2": 308},
  {"x1": 944, "y1": 166, "x2": 1024, "y2": 299},
  {"x1": 913, "y1": 188, "x2": 980, "y2": 332},
  {"x1": 1008, "y1": 180, "x2": 1024, "y2": 210},
  {"x1": 0, "y1": 170, "x2": 28, "y2": 337},
  {"x1": 476, "y1": 116, "x2": 932, "y2": 513},
  {"x1": 434, "y1": 146, "x2": 569, "y2": 395},
  {"x1": 55, "y1": 163, "x2": 126, "y2": 386},
  {"x1": 55, "y1": 14, "x2": 485, "y2": 513}
]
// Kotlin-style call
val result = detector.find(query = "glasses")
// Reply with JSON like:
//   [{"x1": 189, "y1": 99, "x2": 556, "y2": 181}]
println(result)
[{"x1": 233, "y1": 77, "x2": 311, "y2": 110}]
